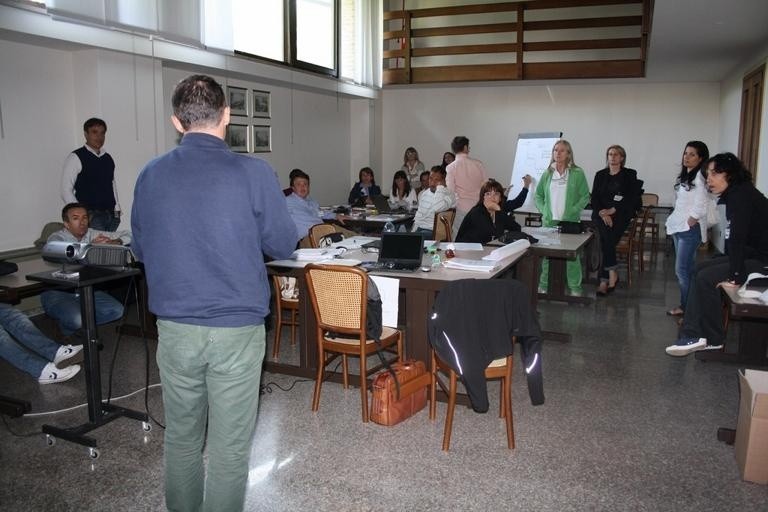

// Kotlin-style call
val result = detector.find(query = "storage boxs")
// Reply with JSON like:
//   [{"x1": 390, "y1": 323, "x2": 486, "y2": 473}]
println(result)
[{"x1": 736, "y1": 368, "x2": 768, "y2": 485}]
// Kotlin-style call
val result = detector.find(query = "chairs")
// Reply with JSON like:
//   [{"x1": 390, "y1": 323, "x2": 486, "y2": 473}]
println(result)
[
  {"x1": 303, "y1": 262, "x2": 404, "y2": 424},
  {"x1": 433, "y1": 209, "x2": 455, "y2": 241},
  {"x1": 427, "y1": 277, "x2": 523, "y2": 451},
  {"x1": 631, "y1": 209, "x2": 651, "y2": 271},
  {"x1": 632, "y1": 193, "x2": 659, "y2": 250},
  {"x1": 616, "y1": 213, "x2": 639, "y2": 288},
  {"x1": 309, "y1": 223, "x2": 337, "y2": 248},
  {"x1": 441, "y1": 216, "x2": 451, "y2": 243},
  {"x1": 273, "y1": 273, "x2": 300, "y2": 358}
]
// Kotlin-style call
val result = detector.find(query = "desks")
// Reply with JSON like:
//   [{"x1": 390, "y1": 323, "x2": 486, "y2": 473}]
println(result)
[
  {"x1": 1, "y1": 257, "x2": 59, "y2": 417},
  {"x1": 25, "y1": 266, "x2": 148, "y2": 448},
  {"x1": 264, "y1": 234, "x2": 531, "y2": 408},
  {"x1": 323, "y1": 214, "x2": 414, "y2": 230},
  {"x1": 487, "y1": 227, "x2": 597, "y2": 305}
]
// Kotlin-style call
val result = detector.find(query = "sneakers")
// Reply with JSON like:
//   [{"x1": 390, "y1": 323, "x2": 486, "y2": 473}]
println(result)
[
  {"x1": 54, "y1": 344, "x2": 83, "y2": 364},
  {"x1": 665, "y1": 337, "x2": 707, "y2": 356},
  {"x1": 38, "y1": 362, "x2": 80, "y2": 384},
  {"x1": 667, "y1": 307, "x2": 683, "y2": 315},
  {"x1": 707, "y1": 344, "x2": 723, "y2": 350}
]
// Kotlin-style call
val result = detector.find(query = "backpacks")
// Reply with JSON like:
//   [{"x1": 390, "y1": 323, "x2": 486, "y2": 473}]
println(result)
[{"x1": 328, "y1": 278, "x2": 381, "y2": 339}]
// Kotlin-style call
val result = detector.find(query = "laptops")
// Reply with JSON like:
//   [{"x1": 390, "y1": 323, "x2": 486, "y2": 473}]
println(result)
[{"x1": 371, "y1": 232, "x2": 424, "y2": 272}]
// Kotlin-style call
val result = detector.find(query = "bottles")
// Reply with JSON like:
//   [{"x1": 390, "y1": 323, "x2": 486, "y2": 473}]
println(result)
[{"x1": 383, "y1": 218, "x2": 440, "y2": 272}]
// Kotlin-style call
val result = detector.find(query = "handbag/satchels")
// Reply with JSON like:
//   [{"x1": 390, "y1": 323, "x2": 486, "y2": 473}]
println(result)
[
  {"x1": 498, "y1": 231, "x2": 538, "y2": 244},
  {"x1": 370, "y1": 361, "x2": 431, "y2": 426},
  {"x1": 319, "y1": 232, "x2": 344, "y2": 249},
  {"x1": 558, "y1": 221, "x2": 584, "y2": 234}
]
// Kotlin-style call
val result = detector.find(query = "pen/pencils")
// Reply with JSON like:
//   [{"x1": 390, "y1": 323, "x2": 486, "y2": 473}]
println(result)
[{"x1": 522, "y1": 175, "x2": 528, "y2": 179}]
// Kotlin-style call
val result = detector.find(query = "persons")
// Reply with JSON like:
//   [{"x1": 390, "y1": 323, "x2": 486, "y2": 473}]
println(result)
[
  {"x1": 286, "y1": 173, "x2": 353, "y2": 240},
  {"x1": 455, "y1": 180, "x2": 521, "y2": 245},
  {"x1": 282, "y1": 169, "x2": 302, "y2": 197},
  {"x1": 0, "y1": 304, "x2": 84, "y2": 385},
  {"x1": 41, "y1": 203, "x2": 132, "y2": 336},
  {"x1": 130, "y1": 76, "x2": 299, "y2": 512},
  {"x1": 489, "y1": 174, "x2": 531, "y2": 213},
  {"x1": 591, "y1": 146, "x2": 645, "y2": 296},
  {"x1": 446, "y1": 136, "x2": 486, "y2": 242},
  {"x1": 665, "y1": 152, "x2": 768, "y2": 357},
  {"x1": 348, "y1": 147, "x2": 454, "y2": 242},
  {"x1": 533, "y1": 141, "x2": 591, "y2": 297},
  {"x1": 60, "y1": 118, "x2": 123, "y2": 232},
  {"x1": 665, "y1": 141, "x2": 710, "y2": 316}
]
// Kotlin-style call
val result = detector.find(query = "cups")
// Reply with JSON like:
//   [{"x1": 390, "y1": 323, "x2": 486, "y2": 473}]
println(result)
[
  {"x1": 552, "y1": 226, "x2": 562, "y2": 245},
  {"x1": 445, "y1": 245, "x2": 456, "y2": 258}
]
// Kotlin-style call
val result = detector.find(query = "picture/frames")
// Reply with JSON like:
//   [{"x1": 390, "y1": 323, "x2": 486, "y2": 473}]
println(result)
[
  {"x1": 252, "y1": 89, "x2": 271, "y2": 118},
  {"x1": 227, "y1": 85, "x2": 248, "y2": 117},
  {"x1": 227, "y1": 123, "x2": 249, "y2": 154},
  {"x1": 252, "y1": 125, "x2": 272, "y2": 152}
]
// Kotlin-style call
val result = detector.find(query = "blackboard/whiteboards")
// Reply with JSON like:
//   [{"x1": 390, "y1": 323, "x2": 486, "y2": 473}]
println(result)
[{"x1": 507, "y1": 132, "x2": 563, "y2": 214}]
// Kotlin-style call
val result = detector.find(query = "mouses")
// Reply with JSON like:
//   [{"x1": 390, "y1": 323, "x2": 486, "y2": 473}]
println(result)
[{"x1": 421, "y1": 265, "x2": 430, "y2": 272}]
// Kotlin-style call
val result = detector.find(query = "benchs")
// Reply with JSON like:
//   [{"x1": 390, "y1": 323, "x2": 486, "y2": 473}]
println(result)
[{"x1": 694, "y1": 239, "x2": 767, "y2": 364}]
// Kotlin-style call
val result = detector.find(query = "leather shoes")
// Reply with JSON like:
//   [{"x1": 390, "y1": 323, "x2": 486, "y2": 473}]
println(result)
[
  {"x1": 608, "y1": 277, "x2": 618, "y2": 292},
  {"x1": 595, "y1": 291, "x2": 606, "y2": 297}
]
